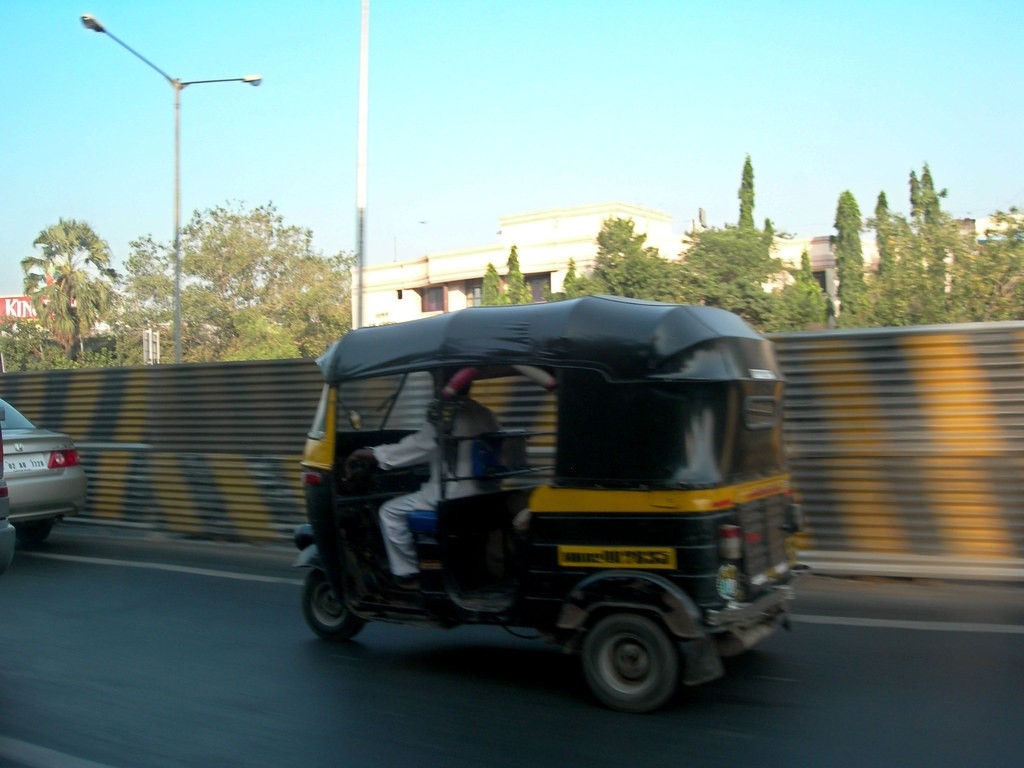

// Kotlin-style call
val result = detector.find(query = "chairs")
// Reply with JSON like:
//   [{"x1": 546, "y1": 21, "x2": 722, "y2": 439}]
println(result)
[{"x1": 407, "y1": 429, "x2": 493, "y2": 542}]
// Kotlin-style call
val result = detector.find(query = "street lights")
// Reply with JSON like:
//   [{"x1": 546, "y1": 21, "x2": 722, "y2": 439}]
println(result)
[{"x1": 82, "y1": 15, "x2": 264, "y2": 363}]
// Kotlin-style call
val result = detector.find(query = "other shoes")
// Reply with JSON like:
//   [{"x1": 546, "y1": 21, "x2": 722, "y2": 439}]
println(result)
[{"x1": 373, "y1": 570, "x2": 421, "y2": 591}]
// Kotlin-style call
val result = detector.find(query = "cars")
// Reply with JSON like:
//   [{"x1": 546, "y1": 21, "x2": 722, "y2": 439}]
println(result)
[{"x1": 0, "y1": 400, "x2": 84, "y2": 550}]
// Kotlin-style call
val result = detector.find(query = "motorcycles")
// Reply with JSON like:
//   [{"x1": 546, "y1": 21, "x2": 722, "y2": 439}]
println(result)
[{"x1": 290, "y1": 296, "x2": 807, "y2": 713}]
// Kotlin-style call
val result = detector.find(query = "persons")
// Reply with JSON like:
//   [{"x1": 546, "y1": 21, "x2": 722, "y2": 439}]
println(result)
[{"x1": 347, "y1": 370, "x2": 496, "y2": 591}]
